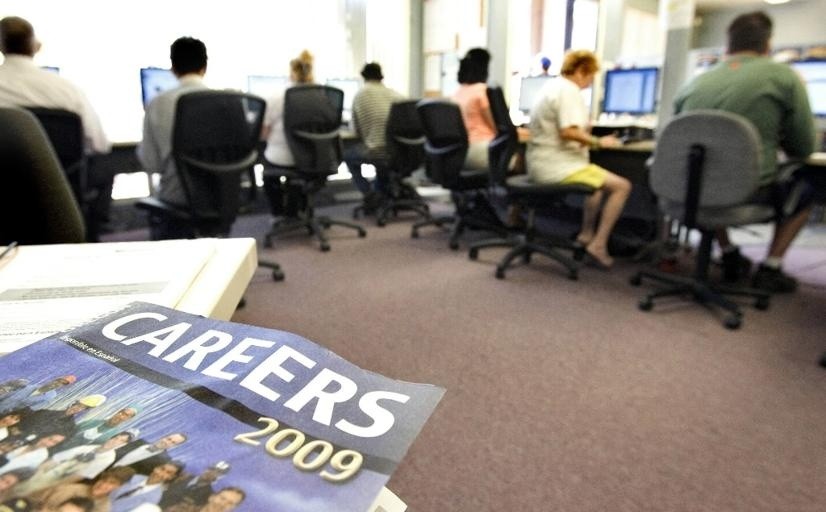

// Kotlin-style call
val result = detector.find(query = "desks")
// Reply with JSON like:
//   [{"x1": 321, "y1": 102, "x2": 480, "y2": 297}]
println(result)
[{"x1": 590, "y1": 124, "x2": 826, "y2": 368}]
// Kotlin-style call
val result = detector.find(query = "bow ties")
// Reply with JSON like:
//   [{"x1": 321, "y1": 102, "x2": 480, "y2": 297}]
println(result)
[{"x1": 146, "y1": 447, "x2": 155, "y2": 453}]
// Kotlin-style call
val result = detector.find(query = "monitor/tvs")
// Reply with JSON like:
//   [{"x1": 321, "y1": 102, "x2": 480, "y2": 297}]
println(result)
[
  {"x1": 248, "y1": 73, "x2": 290, "y2": 102},
  {"x1": 601, "y1": 64, "x2": 660, "y2": 116},
  {"x1": 323, "y1": 76, "x2": 364, "y2": 120},
  {"x1": 777, "y1": 56, "x2": 826, "y2": 116},
  {"x1": 139, "y1": 65, "x2": 180, "y2": 109},
  {"x1": 516, "y1": 74, "x2": 560, "y2": 115}
]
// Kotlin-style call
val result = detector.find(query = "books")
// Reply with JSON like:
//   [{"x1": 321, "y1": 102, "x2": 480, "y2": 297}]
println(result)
[{"x1": 0, "y1": 237, "x2": 259, "y2": 358}]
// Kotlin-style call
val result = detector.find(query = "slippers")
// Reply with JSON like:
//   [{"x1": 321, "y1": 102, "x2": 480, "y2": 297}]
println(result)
[{"x1": 505, "y1": 219, "x2": 526, "y2": 231}]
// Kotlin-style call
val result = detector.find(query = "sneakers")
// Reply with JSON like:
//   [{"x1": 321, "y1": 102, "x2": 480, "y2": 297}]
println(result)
[
  {"x1": 751, "y1": 265, "x2": 800, "y2": 294},
  {"x1": 720, "y1": 248, "x2": 752, "y2": 284}
]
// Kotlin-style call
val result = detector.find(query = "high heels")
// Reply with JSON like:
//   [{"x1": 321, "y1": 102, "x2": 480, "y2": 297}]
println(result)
[
  {"x1": 584, "y1": 244, "x2": 614, "y2": 273},
  {"x1": 570, "y1": 231, "x2": 596, "y2": 261}
]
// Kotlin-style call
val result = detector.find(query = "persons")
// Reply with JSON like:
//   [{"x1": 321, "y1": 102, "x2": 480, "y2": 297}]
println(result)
[
  {"x1": 449, "y1": 48, "x2": 530, "y2": 229},
  {"x1": 673, "y1": 10, "x2": 822, "y2": 294},
  {"x1": 524, "y1": 51, "x2": 633, "y2": 267},
  {"x1": 0, "y1": 371, "x2": 247, "y2": 512},
  {"x1": 343, "y1": 62, "x2": 404, "y2": 200},
  {"x1": 135, "y1": 36, "x2": 220, "y2": 240},
  {"x1": 256, "y1": 50, "x2": 324, "y2": 230},
  {"x1": 0, "y1": 16, "x2": 110, "y2": 238}
]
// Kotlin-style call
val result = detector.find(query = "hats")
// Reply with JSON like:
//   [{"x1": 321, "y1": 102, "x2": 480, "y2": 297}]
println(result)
[
  {"x1": 124, "y1": 428, "x2": 139, "y2": 437},
  {"x1": 99, "y1": 465, "x2": 135, "y2": 482},
  {"x1": 207, "y1": 460, "x2": 231, "y2": 474},
  {"x1": 52, "y1": 375, "x2": 76, "y2": 385},
  {"x1": 0, "y1": 498, "x2": 29, "y2": 511},
  {"x1": 125, "y1": 403, "x2": 144, "y2": 417},
  {"x1": 75, "y1": 394, "x2": 105, "y2": 408}
]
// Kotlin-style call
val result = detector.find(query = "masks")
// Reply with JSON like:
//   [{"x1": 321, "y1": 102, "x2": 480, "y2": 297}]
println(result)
[{"x1": 75, "y1": 422, "x2": 116, "y2": 443}]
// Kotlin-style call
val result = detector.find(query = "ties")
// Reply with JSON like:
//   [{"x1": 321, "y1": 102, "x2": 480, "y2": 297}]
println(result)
[
  {"x1": 114, "y1": 486, "x2": 143, "y2": 499},
  {"x1": 185, "y1": 484, "x2": 196, "y2": 491}
]
